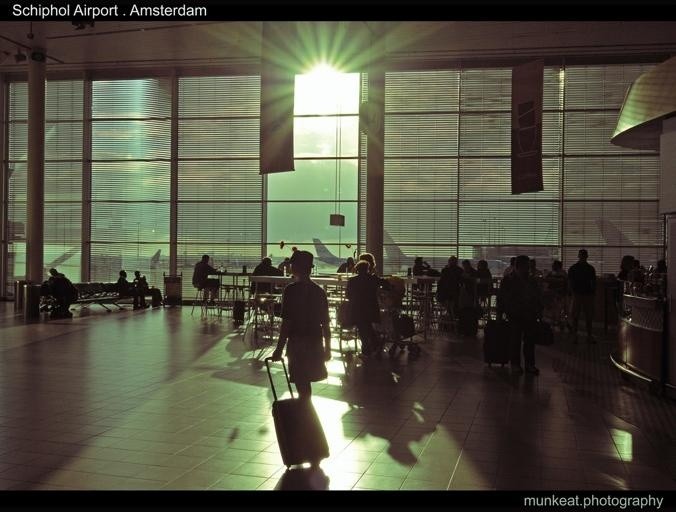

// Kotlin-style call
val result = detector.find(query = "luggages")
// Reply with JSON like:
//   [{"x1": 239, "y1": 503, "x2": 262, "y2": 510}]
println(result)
[
  {"x1": 483, "y1": 319, "x2": 514, "y2": 368},
  {"x1": 264, "y1": 357, "x2": 329, "y2": 468},
  {"x1": 233, "y1": 300, "x2": 244, "y2": 320}
]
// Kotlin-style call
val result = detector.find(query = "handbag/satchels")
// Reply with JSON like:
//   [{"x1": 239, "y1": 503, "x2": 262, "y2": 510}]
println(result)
[
  {"x1": 401, "y1": 314, "x2": 415, "y2": 333},
  {"x1": 535, "y1": 317, "x2": 554, "y2": 345},
  {"x1": 336, "y1": 298, "x2": 354, "y2": 329}
]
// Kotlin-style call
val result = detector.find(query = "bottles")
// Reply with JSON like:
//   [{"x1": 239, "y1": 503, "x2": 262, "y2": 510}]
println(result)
[
  {"x1": 407, "y1": 267, "x2": 412, "y2": 276},
  {"x1": 220, "y1": 263, "x2": 224, "y2": 272},
  {"x1": 543, "y1": 269, "x2": 548, "y2": 278},
  {"x1": 242, "y1": 265, "x2": 247, "y2": 273}
]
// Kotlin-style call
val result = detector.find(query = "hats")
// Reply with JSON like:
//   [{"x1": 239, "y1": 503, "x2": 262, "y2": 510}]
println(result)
[{"x1": 288, "y1": 250, "x2": 315, "y2": 268}]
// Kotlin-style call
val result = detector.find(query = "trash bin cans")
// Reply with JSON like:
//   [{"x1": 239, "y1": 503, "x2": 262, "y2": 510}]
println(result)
[
  {"x1": 21, "y1": 284, "x2": 41, "y2": 324},
  {"x1": 15, "y1": 281, "x2": 30, "y2": 312}
]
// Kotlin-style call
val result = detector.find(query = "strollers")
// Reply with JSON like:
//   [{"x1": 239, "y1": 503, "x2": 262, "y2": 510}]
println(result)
[{"x1": 371, "y1": 303, "x2": 422, "y2": 359}]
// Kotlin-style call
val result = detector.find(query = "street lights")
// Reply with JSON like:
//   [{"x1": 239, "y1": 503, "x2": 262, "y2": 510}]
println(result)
[
  {"x1": 225, "y1": 237, "x2": 232, "y2": 266},
  {"x1": 483, "y1": 218, "x2": 487, "y2": 243},
  {"x1": 182, "y1": 235, "x2": 191, "y2": 268},
  {"x1": 135, "y1": 221, "x2": 140, "y2": 261},
  {"x1": 491, "y1": 215, "x2": 498, "y2": 244}
]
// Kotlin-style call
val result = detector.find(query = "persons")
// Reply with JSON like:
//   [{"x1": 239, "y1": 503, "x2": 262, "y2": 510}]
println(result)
[{"x1": 39, "y1": 248, "x2": 668, "y2": 403}]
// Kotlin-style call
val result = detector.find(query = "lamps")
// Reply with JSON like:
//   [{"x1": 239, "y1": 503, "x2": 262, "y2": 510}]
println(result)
[{"x1": 330, "y1": 75, "x2": 345, "y2": 226}]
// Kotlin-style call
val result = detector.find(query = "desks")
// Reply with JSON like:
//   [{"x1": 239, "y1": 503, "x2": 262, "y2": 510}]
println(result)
[
  {"x1": 208, "y1": 271, "x2": 253, "y2": 321},
  {"x1": 413, "y1": 275, "x2": 498, "y2": 328},
  {"x1": 249, "y1": 275, "x2": 417, "y2": 349}
]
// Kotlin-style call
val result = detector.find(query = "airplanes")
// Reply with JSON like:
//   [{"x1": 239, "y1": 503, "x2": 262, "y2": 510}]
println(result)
[
  {"x1": 123, "y1": 248, "x2": 164, "y2": 275},
  {"x1": 312, "y1": 228, "x2": 511, "y2": 282},
  {"x1": 594, "y1": 218, "x2": 666, "y2": 270}
]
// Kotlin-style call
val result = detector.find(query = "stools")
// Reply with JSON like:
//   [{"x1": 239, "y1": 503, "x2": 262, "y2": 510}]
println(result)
[{"x1": 192, "y1": 283, "x2": 577, "y2": 346}]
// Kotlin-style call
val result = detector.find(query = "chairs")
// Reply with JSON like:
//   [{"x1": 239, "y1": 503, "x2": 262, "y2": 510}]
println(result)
[{"x1": 42, "y1": 282, "x2": 144, "y2": 312}]
